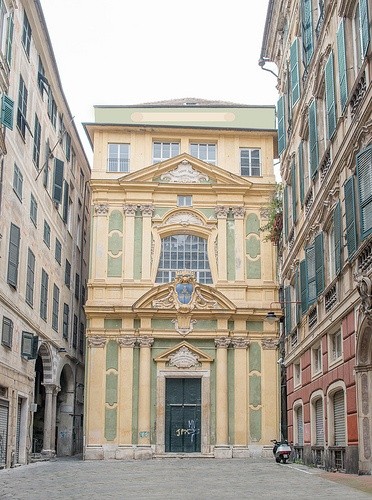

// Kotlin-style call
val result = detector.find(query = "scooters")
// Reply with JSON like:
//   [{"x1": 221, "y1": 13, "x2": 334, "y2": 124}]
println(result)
[{"x1": 271, "y1": 440, "x2": 293, "y2": 463}]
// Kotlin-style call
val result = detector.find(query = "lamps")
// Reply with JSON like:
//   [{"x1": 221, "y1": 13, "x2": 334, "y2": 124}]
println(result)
[
  {"x1": 266, "y1": 301, "x2": 303, "y2": 324},
  {"x1": 38, "y1": 339, "x2": 66, "y2": 352}
]
